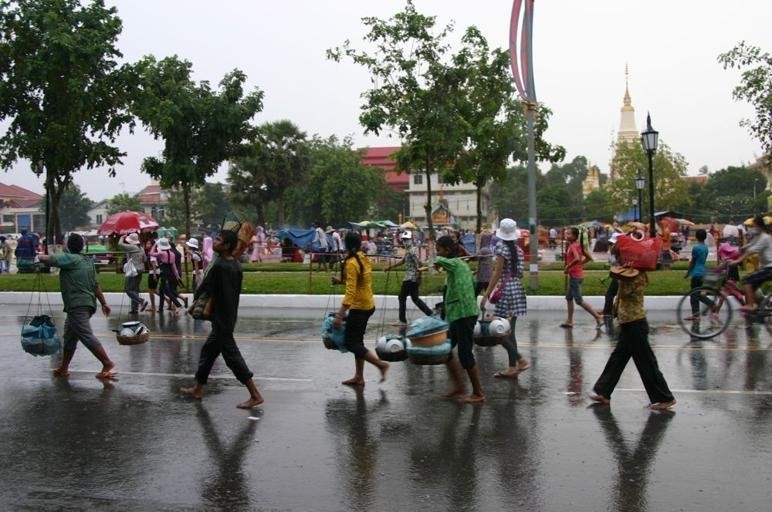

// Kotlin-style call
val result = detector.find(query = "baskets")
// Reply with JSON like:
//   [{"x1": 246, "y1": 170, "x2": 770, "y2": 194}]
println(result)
[
  {"x1": 374, "y1": 347, "x2": 410, "y2": 363},
  {"x1": 116, "y1": 331, "x2": 151, "y2": 346},
  {"x1": 20, "y1": 340, "x2": 62, "y2": 357},
  {"x1": 472, "y1": 334, "x2": 515, "y2": 348},
  {"x1": 406, "y1": 351, "x2": 454, "y2": 366},
  {"x1": 321, "y1": 332, "x2": 353, "y2": 351}
]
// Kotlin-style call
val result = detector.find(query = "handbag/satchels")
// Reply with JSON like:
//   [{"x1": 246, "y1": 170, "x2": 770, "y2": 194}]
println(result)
[
  {"x1": 122, "y1": 258, "x2": 138, "y2": 278},
  {"x1": 617, "y1": 224, "x2": 659, "y2": 271}
]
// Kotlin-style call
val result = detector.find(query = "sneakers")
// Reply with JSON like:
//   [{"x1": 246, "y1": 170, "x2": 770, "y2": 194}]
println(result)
[{"x1": 127, "y1": 295, "x2": 190, "y2": 316}]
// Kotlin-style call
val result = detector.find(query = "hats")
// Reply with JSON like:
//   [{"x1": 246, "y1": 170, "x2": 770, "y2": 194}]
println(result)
[
  {"x1": 156, "y1": 238, "x2": 174, "y2": 251},
  {"x1": 494, "y1": 219, "x2": 523, "y2": 243},
  {"x1": 401, "y1": 229, "x2": 414, "y2": 239},
  {"x1": 185, "y1": 236, "x2": 201, "y2": 251},
  {"x1": 124, "y1": 232, "x2": 141, "y2": 246}
]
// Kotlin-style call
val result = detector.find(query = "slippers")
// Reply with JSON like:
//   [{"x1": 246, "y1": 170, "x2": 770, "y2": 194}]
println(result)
[
  {"x1": 516, "y1": 362, "x2": 533, "y2": 371},
  {"x1": 607, "y1": 260, "x2": 640, "y2": 281},
  {"x1": 52, "y1": 368, "x2": 72, "y2": 376},
  {"x1": 492, "y1": 368, "x2": 521, "y2": 379},
  {"x1": 95, "y1": 370, "x2": 122, "y2": 379},
  {"x1": 558, "y1": 320, "x2": 608, "y2": 329}
]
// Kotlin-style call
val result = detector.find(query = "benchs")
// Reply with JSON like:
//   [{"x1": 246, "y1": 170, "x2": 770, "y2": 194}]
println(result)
[{"x1": 82, "y1": 253, "x2": 128, "y2": 273}]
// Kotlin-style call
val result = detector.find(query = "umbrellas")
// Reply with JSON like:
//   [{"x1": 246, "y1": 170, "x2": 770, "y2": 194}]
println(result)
[{"x1": 98, "y1": 210, "x2": 159, "y2": 235}]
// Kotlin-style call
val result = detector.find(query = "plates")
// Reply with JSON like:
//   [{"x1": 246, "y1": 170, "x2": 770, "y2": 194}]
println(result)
[
  {"x1": 378, "y1": 337, "x2": 405, "y2": 352},
  {"x1": 471, "y1": 316, "x2": 511, "y2": 337},
  {"x1": 116, "y1": 320, "x2": 147, "y2": 335}
]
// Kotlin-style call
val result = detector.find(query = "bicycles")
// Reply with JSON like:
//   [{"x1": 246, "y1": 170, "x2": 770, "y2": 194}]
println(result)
[{"x1": 677, "y1": 243, "x2": 771, "y2": 342}]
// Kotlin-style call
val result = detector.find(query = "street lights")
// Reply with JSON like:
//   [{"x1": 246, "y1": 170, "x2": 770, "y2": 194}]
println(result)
[{"x1": 632, "y1": 111, "x2": 660, "y2": 243}]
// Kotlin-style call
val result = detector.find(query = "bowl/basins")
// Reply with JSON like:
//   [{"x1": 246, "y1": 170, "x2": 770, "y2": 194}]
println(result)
[{"x1": 406, "y1": 321, "x2": 448, "y2": 346}]
